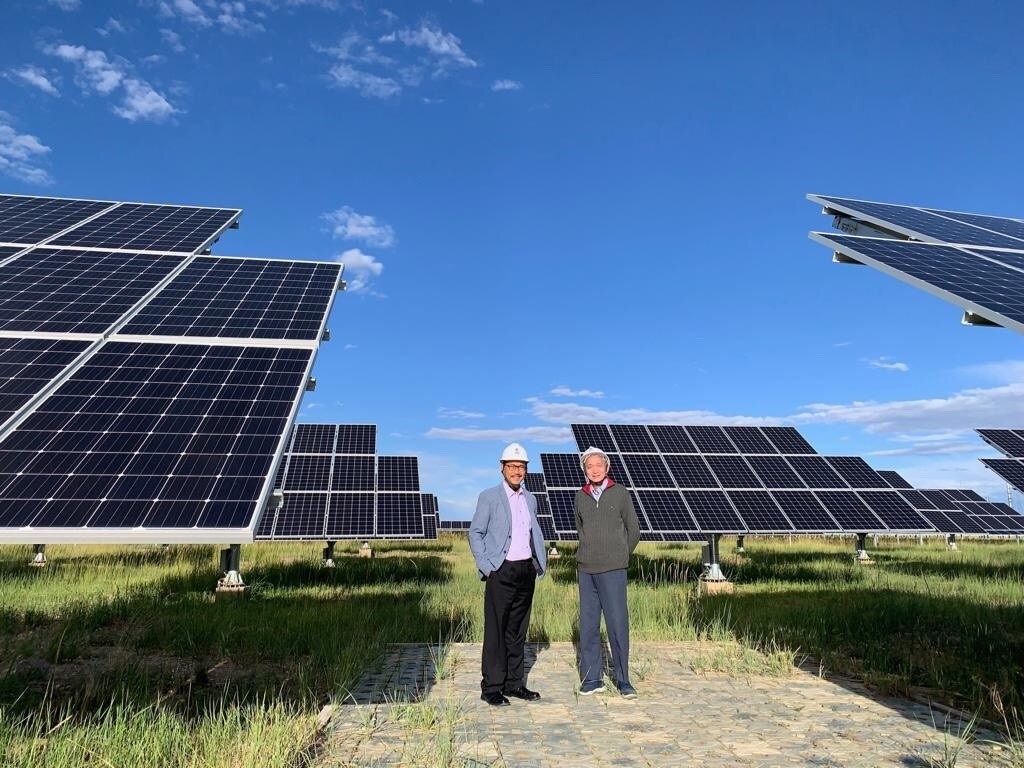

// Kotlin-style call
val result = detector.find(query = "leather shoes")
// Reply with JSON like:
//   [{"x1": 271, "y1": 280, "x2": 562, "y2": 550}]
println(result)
[
  {"x1": 481, "y1": 691, "x2": 509, "y2": 706},
  {"x1": 501, "y1": 686, "x2": 541, "y2": 701}
]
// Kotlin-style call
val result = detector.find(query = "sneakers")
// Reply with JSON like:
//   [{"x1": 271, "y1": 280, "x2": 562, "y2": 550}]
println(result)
[
  {"x1": 578, "y1": 680, "x2": 608, "y2": 694},
  {"x1": 616, "y1": 678, "x2": 637, "y2": 700}
]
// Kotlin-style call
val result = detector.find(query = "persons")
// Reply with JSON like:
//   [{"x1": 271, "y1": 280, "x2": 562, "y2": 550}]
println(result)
[
  {"x1": 572, "y1": 446, "x2": 637, "y2": 699},
  {"x1": 468, "y1": 442, "x2": 546, "y2": 706}
]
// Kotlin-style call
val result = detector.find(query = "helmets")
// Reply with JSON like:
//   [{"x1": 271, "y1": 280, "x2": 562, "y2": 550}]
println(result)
[
  {"x1": 580, "y1": 446, "x2": 610, "y2": 472},
  {"x1": 499, "y1": 443, "x2": 529, "y2": 462}
]
